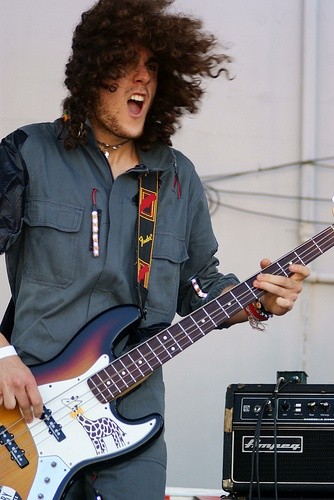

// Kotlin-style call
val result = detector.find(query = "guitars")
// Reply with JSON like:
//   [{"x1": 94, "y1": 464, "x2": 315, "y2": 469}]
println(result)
[{"x1": 0, "y1": 223, "x2": 334, "y2": 500}]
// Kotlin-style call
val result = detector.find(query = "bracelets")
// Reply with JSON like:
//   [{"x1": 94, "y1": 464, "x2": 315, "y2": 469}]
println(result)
[
  {"x1": 243, "y1": 298, "x2": 275, "y2": 331},
  {"x1": 1, "y1": 345, "x2": 18, "y2": 360}
]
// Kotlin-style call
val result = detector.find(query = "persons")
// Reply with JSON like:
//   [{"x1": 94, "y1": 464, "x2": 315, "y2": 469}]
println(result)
[{"x1": 1, "y1": 0, "x2": 313, "y2": 500}]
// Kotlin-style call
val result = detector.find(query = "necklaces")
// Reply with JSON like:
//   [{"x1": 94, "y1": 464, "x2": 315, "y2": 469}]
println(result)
[{"x1": 94, "y1": 139, "x2": 131, "y2": 159}]
